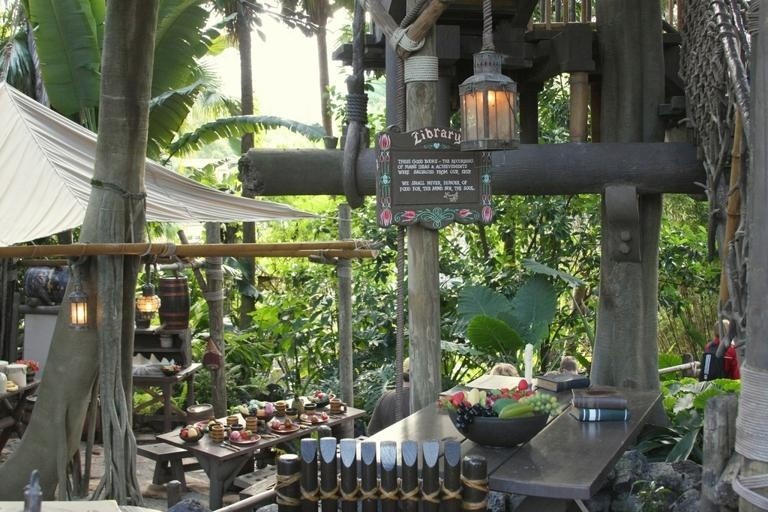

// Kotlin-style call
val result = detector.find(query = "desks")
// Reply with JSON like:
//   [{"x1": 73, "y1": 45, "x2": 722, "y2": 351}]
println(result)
[
  {"x1": 153, "y1": 394, "x2": 369, "y2": 510},
  {"x1": 2, "y1": 500, "x2": 158, "y2": 510},
  {"x1": 1, "y1": 374, "x2": 42, "y2": 444},
  {"x1": 336, "y1": 377, "x2": 664, "y2": 510}
]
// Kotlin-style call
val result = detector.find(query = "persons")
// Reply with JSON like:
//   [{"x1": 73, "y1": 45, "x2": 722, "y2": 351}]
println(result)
[
  {"x1": 559, "y1": 355, "x2": 578, "y2": 376},
  {"x1": 699, "y1": 319, "x2": 740, "y2": 382},
  {"x1": 490, "y1": 361, "x2": 519, "y2": 376},
  {"x1": 680, "y1": 351, "x2": 698, "y2": 380}
]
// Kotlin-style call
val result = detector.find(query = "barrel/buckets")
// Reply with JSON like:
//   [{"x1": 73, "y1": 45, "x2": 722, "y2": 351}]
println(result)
[
  {"x1": 5, "y1": 364, "x2": 28, "y2": 386},
  {"x1": 23, "y1": 267, "x2": 69, "y2": 300},
  {"x1": 21, "y1": 395, "x2": 37, "y2": 441},
  {"x1": 159, "y1": 276, "x2": 189, "y2": 329}
]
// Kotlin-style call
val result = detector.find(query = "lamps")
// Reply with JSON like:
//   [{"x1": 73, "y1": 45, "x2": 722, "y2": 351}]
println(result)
[
  {"x1": 459, "y1": 0, "x2": 521, "y2": 152},
  {"x1": 136, "y1": 253, "x2": 162, "y2": 318},
  {"x1": 66, "y1": 280, "x2": 90, "y2": 332}
]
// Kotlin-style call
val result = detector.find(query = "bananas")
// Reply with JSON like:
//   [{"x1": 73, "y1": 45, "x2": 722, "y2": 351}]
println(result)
[
  {"x1": 5, "y1": 380, "x2": 19, "y2": 392},
  {"x1": 497, "y1": 402, "x2": 535, "y2": 419}
]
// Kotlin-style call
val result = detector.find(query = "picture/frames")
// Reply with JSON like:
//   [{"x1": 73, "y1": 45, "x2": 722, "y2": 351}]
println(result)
[{"x1": 374, "y1": 126, "x2": 492, "y2": 230}]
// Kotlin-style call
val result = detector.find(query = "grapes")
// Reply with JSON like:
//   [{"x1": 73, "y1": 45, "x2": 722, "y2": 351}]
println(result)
[
  {"x1": 518, "y1": 390, "x2": 560, "y2": 419},
  {"x1": 455, "y1": 402, "x2": 497, "y2": 429}
]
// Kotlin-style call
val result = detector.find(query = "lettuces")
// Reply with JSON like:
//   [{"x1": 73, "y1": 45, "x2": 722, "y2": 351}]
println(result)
[{"x1": 133, "y1": 392, "x2": 164, "y2": 416}]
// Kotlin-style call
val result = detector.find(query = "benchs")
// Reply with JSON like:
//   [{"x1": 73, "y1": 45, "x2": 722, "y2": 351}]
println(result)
[
  {"x1": 239, "y1": 473, "x2": 277, "y2": 498},
  {"x1": 135, "y1": 442, "x2": 272, "y2": 493}
]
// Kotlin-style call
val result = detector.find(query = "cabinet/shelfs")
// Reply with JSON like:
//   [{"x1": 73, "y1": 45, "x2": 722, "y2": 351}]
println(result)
[{"x1": 132, "y1": 324, "x2": 203, "y2": 434}]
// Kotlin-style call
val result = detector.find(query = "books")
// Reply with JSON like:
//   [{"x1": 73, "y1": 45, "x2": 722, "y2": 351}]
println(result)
[
  {"x1": 570, "y1": 386, "x2": 628, "y2": 410},
  {"x1": 534, "y1": 370, "x2": 591, "y2": 394},
  {"x1": 567, "y1": 401, "x2": 632, "y2": 422}
]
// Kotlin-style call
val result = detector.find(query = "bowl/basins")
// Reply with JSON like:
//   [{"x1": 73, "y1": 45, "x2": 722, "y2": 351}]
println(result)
[
  {"x1": 161, "y1": 369, "x2": 180, "y2": 376},
  {"x1": 180, "y1": 428, "x2": 204, "y2": 443},
  {"x1": 240, "y1": 405, "x2": 275, "y2": 425},
  {"x1": 447, "y1": 406, "x2": 551, "y2": 449},
  {"x1": 307, "y1": 393, "x2": 336, "y2": 408}
]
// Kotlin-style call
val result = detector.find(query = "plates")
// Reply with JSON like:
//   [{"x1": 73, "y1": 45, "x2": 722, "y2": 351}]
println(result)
[
  {"x1": 229, "y1": 432, "x2": 261, "y2": 445},
  {"x1": 270, "y1": 422, "x2": 301, "y2": 433},
  {"x1": 194, "y1": 420, "x2": 223, "y2": 434},
  {"x1": 300, "y1": 412, "x2": 329, "y2": 422}
]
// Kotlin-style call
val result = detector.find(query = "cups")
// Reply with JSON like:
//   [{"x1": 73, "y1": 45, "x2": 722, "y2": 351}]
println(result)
[
  {"x1": 330, "y1": 399, "x2": 347, "y2": 415},
  {"x1": 227, "y1": 416, "x2": 238, "y2": 435},
  {"x1": 274, "y1": 401, "x2": 286, "y2": 417},
  {"x1": 246, "y1": 417, "x2": 258, "y2": 433},
  {"x1": 212, "y1": 425, "x2": 231, "y2": 443}
]
